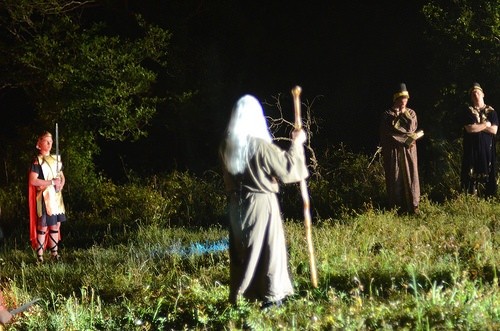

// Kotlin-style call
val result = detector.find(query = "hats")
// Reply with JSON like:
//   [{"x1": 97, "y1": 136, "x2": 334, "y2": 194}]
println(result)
[
  {"x1": 468, "y1": 82, "x2": 482, "y2": 95},
  {"x1": 393, "y1": 83, "x2": 410, "y2": 102}
]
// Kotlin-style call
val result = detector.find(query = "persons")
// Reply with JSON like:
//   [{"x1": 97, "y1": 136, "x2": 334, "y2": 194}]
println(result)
[
  {"x1": 1, "y1": 300, "x2": 17, "y2": 326},
  {"x1": 222, "y1": 94, "x2": 307, "y2": 309},
  {"x1": 27, "y1": 131, "x2": 65, "y2": 262},
  {"x1": 462, "y1": 82, "x2": 498, "y2": 199},
  {"x1": 384, "y1": 83, "x2": 419, "y2": 216}
]
